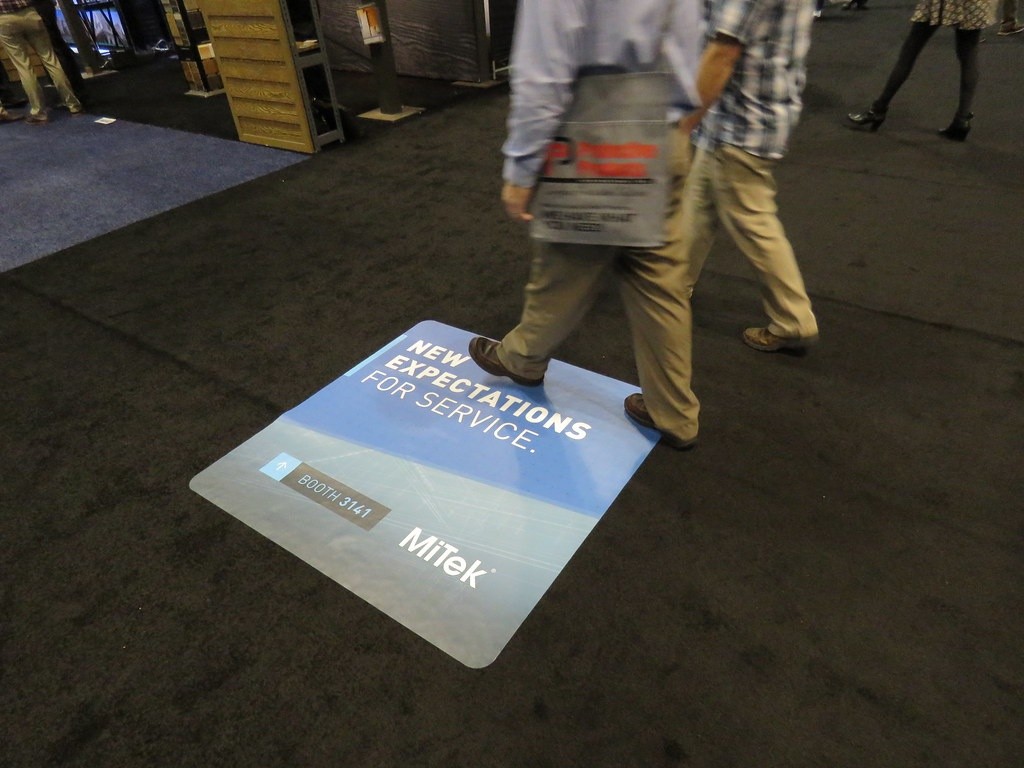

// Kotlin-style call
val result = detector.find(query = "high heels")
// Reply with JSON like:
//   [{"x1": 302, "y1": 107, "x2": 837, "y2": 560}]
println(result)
[
  {"x1": 848, "y1": 101, "x2": 889, "y2": 132},
  {"x1": 937, "y1": 112, "x2": 974, "y2": 142}
]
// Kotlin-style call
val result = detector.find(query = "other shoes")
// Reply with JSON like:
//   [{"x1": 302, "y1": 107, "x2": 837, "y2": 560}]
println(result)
[
  {"x1": 813, "y1": 8, "x2": 822, "y2": 18},
  {"x1": 0, "y1": 112, "x2": 24, "y2": 122},
  {"x1": 25, "y1": 114, "x2": 47, "y2": 125},
  {"x1": 468, "y1": 336, "x2": 545, "y2": 387},
  {"x1": 624, "y1": 393, "x2": 697, "y2": 452},
  {"x1": 997, "y1": 21, "x2": 1024, "y2": 36}
]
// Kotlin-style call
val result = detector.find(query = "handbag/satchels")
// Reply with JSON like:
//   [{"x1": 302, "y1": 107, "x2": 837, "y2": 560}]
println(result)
[{"x1": 529, "y1": 70, "x2": 667, "y2": 249}]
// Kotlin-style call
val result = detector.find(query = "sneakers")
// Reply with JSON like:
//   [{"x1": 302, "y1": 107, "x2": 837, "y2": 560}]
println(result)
[{"x1": 742, "y1": 327, "x2": 821, "y2": 352}]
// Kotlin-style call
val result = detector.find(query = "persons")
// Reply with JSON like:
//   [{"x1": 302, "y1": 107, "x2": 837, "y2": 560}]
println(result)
[
  {"x1": 0, "y1": 0, "x2": 83, "y2": 124},
  {"x1": 847, "y1": 0, "x2": 1024, "y2": 142},
  {"x1": 468, "y1": 0, "x2": 818, "y2": 451}
]
